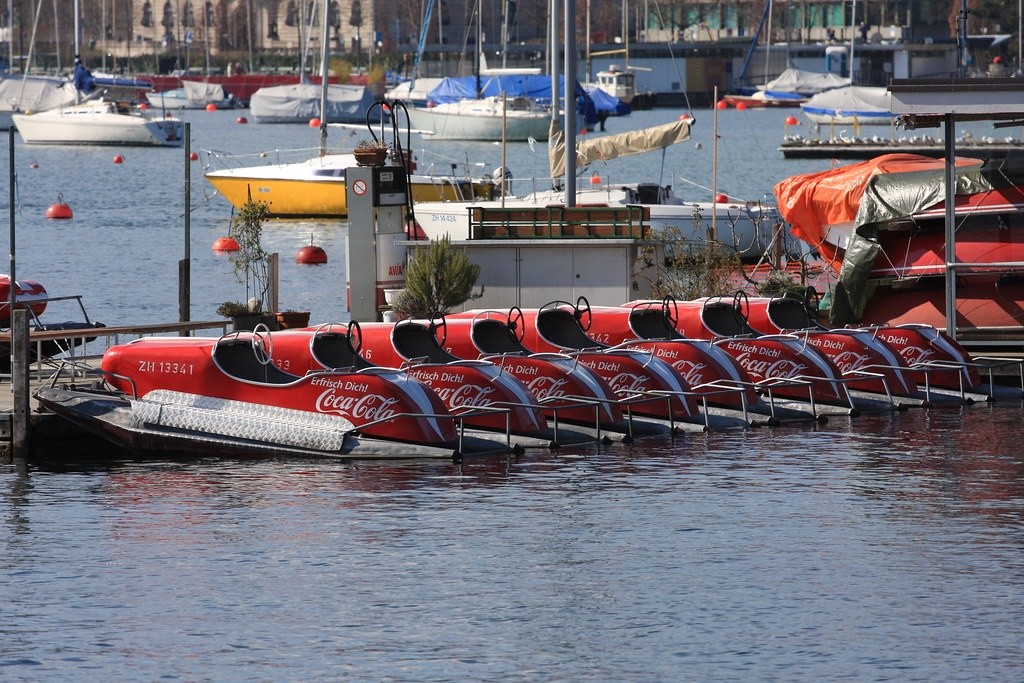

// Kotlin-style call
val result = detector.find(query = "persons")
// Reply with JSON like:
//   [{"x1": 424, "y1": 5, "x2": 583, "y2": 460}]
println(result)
[
  {"x1": 859, "y1": 22, "x2": 867, "y2": 40},
  {"x1": 826, "y1": 28, "x2": 837, "y2": 41}
]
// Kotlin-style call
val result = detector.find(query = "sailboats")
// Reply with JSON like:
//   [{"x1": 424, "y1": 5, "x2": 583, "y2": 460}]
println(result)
[
  {"x1": 203, "y1": 0, "x2": 513, "y2": 215},
  {"x1": 411, "y1": 1, "x2": 802, "y2": 263},
  {"x1": 0, "y1": 0, "x2": 633, "y2": 148}
]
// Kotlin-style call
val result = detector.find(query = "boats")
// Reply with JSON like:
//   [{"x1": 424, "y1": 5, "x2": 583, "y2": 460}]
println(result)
[{"x1": 31, "y1": 287, "x2": 990, "y2": 459}]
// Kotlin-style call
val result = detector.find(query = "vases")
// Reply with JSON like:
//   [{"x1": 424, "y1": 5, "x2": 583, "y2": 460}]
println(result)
[{"x1": 381, "y1": 287, "x2": 411, "y2": 324}]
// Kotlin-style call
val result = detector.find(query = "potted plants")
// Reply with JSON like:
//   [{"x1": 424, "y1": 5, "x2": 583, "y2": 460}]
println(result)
[
  {"x1": 216, "y1": 198, "x2": 276, "y2": 332},
  {"x1": 392, "y1": 232, "x2": 486, "y2": 323},
  {"x1": 273, "y1": 308, "x2": 312, "y2": 330}
]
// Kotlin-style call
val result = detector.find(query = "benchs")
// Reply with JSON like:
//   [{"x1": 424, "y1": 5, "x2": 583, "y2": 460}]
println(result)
[{"x1": 0, "y1": 321, "x2": 233, "y2": 382}]
